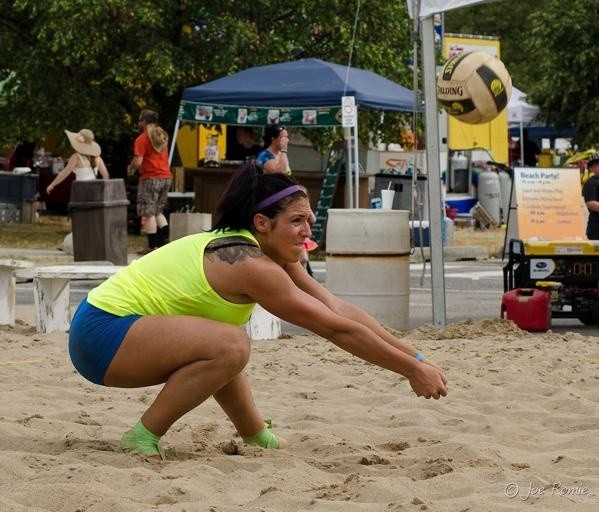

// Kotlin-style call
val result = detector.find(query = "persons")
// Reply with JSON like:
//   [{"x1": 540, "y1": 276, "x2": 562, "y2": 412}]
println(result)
[
  {"x1": 516, "y1": 125, "x2": 542, "y2": 168},
  {"x1": 66, "y1": 161, "x2": 450, "y2": 464},
  {"x1": 580, "y1": 152, "x2": 599, "y2": 242},
  {"x1": 45, "y1": 128, "x2": 110, "y2": 255},
  {"x1": 235, "y1": 125, "x2": 264, "y2": 160},
  {"x1": 256, "y1": 122, "x2": 315, "y2": 281},
  {"x1": 126, "y1": 108, "x2": 171, "y2": 255}
]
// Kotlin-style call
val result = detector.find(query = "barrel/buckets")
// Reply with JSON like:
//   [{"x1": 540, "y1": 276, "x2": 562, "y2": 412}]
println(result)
[
  {"x1": 240, "y1": 303, "x2": 281, "y2": 340},
  {"x1": 325, "y1": 208, "x2": 409, "y2": 331},
  {"x1": 501, "y1": 288, "x2": 551, "y2": 332}
]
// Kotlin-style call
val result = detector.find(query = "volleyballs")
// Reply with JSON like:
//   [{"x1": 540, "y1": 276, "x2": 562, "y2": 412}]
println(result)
[{"x1": 436, "y1": 50, "x2": 512, "y2": 124}]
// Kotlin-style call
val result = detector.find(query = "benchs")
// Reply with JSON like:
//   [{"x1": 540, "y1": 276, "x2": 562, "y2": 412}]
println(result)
[
  {"x1": 0, "y1": 258, "x2": 31, "y2": 326},
  {"x1": 13, "y1": 264, "x2": 294, "y2": 342}
]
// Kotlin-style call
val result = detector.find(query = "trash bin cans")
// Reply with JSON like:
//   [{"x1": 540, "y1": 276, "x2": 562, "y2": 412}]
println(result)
[
  {"x1": 374, "y1": 174, "x2": 428, "y2": 220},
  {"x1": 67, "y1": 178, "x2": 131, "y2": 266}
]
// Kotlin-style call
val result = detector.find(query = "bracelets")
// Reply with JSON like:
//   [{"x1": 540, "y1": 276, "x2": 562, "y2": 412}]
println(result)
[
  {"x1": 279, "y1": 149, "x2": 289, "y2": 155},
  {"x1": 412, "y1": 350, "x2": 427, "y2": 362}
]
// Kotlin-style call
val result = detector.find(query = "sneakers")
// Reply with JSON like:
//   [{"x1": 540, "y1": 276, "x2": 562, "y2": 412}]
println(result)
[{"x1": 135, "y1": 247, "x2": 156, "y2": 256}]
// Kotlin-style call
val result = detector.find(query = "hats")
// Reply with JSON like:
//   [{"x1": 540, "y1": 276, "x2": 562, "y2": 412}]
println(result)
[
  {"x1": 586, "y1": 152, "x2": 599, "y2": 169},
  {"x1": 64, "y1": 128, "x2": 102, "y2": 156},
  {"x1": 146, "y1": 123, "x2": 169, "y2": 153}
]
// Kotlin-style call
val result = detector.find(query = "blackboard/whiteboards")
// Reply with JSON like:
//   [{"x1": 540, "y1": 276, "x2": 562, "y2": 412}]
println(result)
[{"x1": 311, "y1": 140, "x2": 346, "y2": 250}]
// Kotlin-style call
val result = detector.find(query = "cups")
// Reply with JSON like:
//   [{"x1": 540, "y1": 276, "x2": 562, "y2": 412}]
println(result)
[{"x1": 380, "y1": 190, "x2": 395, "y2": 210}]
